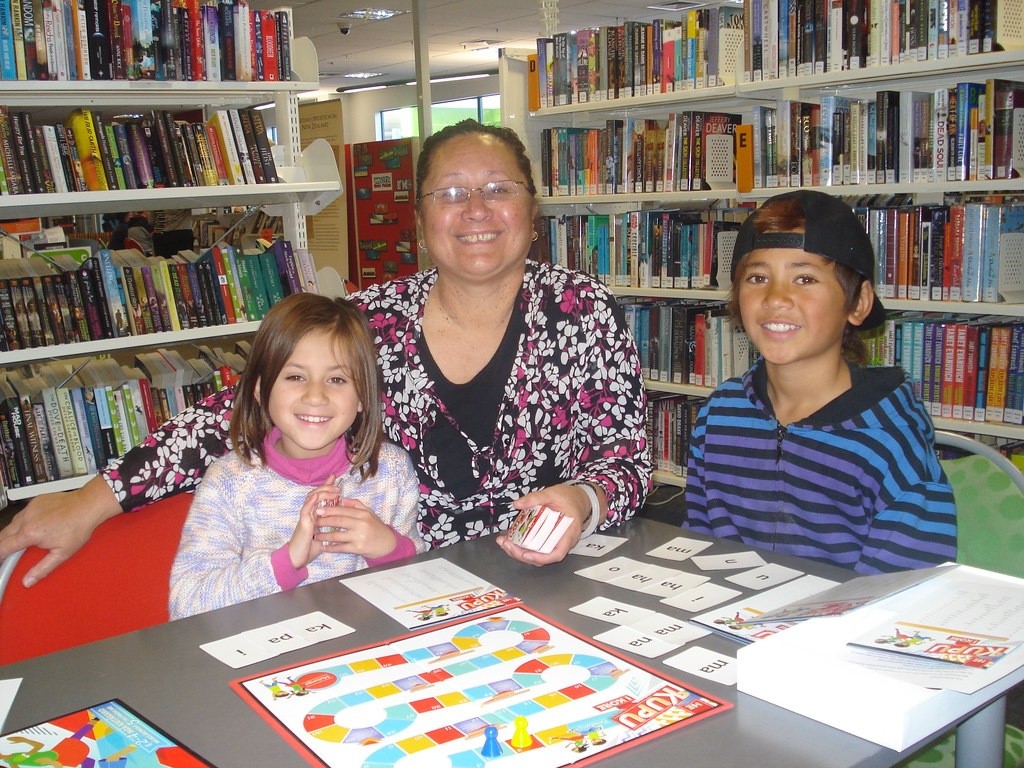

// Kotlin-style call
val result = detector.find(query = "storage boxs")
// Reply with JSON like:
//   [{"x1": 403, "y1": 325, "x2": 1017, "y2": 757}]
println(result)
[{"x1": 736, "y1": 618, "x2": 1024, "y2": 753}]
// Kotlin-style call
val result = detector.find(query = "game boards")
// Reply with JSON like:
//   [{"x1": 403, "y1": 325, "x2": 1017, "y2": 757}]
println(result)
[{"x1": 226, "y1": 601, "x2": 736, "y2": 767}]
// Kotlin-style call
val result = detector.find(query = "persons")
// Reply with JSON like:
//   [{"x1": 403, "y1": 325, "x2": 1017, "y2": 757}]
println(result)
[
  {"x1": 128, "y1": 211, "x2": 154, "y2": 258},
  {"x1": 27, "y1": 301, "x2": 41, "y2": 330},
  {"x1": 0, "y1": 118, "x2": 653, "y2": 589},
  {"x1": 589, "y1": 44, "x2": 595, "y2": 71},
  {"x1": 197, "y1": 298, "x2": 203, "y2": 312},
  {"x1": 73, "y1": 160, "x2": 83, "y2": 177},
  {"x1": 581, "y1": 51, "x2": 585, "y2": 64},
  {"x1": 151, "y1": 299, "x2": 157, "y2": 307},
  {"x1": 234, "y1": 207, "x2": 244, "y2": 214},
  {"x1": 605, "y1": 155, "x2": 613, "y2": 182},
  {"x1": 678, "y1": 190, "x2": 959, "y2": 577},
  {"x1": 16, "y1": 303, "x2": 30, "y2": 331},
  {"x1": 977, "y1": 120, "x2": 985, "y2": 136},
  {"x1": 107, "y1": 212, "x2": 134, "y2": 251},
  {"x1": 148, "y1": 210, "x2": 195, "y2": 258},
  {"x1": 168, "y1": 291, "x2": 424, "y2": 621},
  {"x1": 240, "y1": 304, "x2": 246, "y2": 318},
  {"x1": 913, "y1": 242, "x2": 920, "y2": 258}
]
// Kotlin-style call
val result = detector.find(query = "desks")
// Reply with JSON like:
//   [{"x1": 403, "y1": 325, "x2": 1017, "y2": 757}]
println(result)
[{"x1": 0, "y1": 520, "x2": 1024, "y2": 768}]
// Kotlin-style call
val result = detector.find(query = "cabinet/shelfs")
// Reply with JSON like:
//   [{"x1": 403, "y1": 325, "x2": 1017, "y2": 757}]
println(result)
[
  {"x1": 499, "y1": 0, "x2": 1024, "y2": 489},
  {"x1": 0, "y1": 0, "x2": 346, "y2": 501}
]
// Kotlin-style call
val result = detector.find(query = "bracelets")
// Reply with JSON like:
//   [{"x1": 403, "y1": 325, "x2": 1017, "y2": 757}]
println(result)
[{"x1": 565, "y1": 480, "x2": 600, "y2": 540}]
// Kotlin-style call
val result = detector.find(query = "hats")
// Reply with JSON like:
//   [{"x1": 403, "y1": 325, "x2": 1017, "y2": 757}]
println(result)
[{"x1": 731, "y1": 190, "x2": 885, "y2": 332}]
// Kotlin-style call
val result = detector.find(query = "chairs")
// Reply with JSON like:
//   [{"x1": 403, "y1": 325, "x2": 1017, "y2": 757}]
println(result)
[
  {"x1": 0, "y1": 492, "x2": 195, "y2": 673},
  {"x1": 932, "y1": 431, "x2": 1024, "y2": 580}
]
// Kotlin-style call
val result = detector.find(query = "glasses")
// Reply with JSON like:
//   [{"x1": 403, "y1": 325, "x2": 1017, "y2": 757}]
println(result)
[{"x1": 418, "y1": 180, "x2": 532, "y2": 208}]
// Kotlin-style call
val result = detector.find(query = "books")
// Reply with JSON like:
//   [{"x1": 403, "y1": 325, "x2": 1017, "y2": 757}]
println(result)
[
  {"x1": 0, "y1": 0, "x2": 318, "y2": 490},
  {"x1": 843, "y1": 620, "x2": 1024, "y2": 694},
  {"x1": 527, "y1": 0, "x2": 1024, "y2": 479}
]
